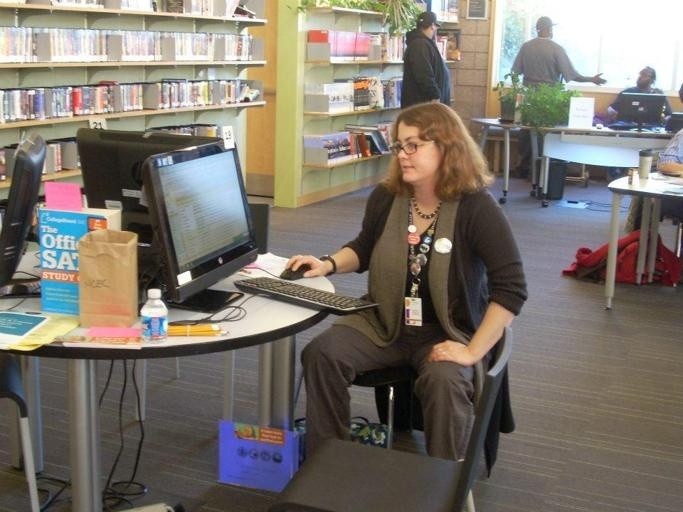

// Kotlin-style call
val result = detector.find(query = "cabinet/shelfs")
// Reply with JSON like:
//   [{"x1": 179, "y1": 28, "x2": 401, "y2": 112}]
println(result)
[
  {"x1": 0, "y1": 1, "x2": 269, "y2": 190},
  {"x1": 302, "y1": 5, "x2": 461, "y2": 170}
]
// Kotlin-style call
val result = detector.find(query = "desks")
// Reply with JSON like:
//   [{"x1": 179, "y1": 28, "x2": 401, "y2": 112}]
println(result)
[
  {"x1": 602, "y1": 172, "x2": 683, "y2": 310},
  {"x1": 0, "y1": 246, "x2": 336, "y2": 512},
  {"x1": 519, "y1": 123, "x2": 677, "y2": 207},
  {"x1": 470, "y1": 117, "x2": 540, "y2": 205}
]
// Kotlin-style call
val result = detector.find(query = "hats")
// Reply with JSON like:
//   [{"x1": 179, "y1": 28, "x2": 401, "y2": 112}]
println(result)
[
  {"x1": 417, "y1": 11, "x2": 439, "y2": 26},
  {"x1": 537, "y1": 16, "x2": 556, "y2": 30}
]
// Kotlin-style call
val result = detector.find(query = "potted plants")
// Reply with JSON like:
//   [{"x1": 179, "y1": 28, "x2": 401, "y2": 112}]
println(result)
[{"x1": 491, "y1": 72, "x2": 532, "y2": 123}]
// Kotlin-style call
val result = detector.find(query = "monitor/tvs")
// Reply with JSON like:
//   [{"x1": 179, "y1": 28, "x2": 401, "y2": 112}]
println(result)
[
  {"x1": 0, "y1": 133, "x2": 47, "y2": 296},
  {"x1": 141, "y1": 140, "x2": 259, "y2": 314},
  {"x1": 617, "y1": 93, "x2": 667, "y2": 132},
  {"x1": 77, "y1": 128, "x2": 224, "y2": 245}
]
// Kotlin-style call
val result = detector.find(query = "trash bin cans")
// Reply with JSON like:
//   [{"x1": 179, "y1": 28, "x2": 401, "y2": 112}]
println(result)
[{"x1": 536, "y1": 156, "x2": 569, "y2": 200}]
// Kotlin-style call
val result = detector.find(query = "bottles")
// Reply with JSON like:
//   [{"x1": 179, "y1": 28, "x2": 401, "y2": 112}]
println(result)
[
  {"x1": 639, "y1": 148, "x2": 653, "y2": 179},
  {"x1": 138, "y1": 288, "x2": 170, "y2": 346},
  {"x1": 367, "y1": 34, "x2": 380, "y2": 62}
]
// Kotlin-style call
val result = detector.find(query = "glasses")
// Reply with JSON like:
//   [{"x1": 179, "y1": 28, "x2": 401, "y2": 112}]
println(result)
[{"x1": 389, "y1": 141, "x2": 435, "y2": 154}]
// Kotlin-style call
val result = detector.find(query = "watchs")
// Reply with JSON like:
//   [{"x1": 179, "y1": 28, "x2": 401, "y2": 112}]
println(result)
[{"x1": 319, "y1": 254, "x2": 337, "y2": 277}]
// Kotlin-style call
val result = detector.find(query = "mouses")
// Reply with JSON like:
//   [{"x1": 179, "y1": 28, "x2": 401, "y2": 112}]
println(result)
[
  {"x1": 596, "y1": 124, "x2": 603, "y2": 129},
  {"x1": 280, "y1": 264, "x2": 310, "y2": 280}
]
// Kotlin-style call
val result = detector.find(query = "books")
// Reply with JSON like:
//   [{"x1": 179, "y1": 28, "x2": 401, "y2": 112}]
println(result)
[
  {"x1": 2, "y1": 79, "x2": 258, "y2": 122},
  {"x1": 304, "y1": 30, "x2": 406, "y2": 166},
  {"x1": 434, "y1": 32, "x2": 460, "y2": 62},
  {"x1": 1, "y1": 124, "x2": 234, "y2": 182},
  {"x1": 29, "y1": 189, "x2": 122, "y2": 316},
  {"x1": 0, "y1": 1, "x2": 255, "y2": 18},
  {"x1": 0, "y1": 27, "x2": 252, "y2": 62}
]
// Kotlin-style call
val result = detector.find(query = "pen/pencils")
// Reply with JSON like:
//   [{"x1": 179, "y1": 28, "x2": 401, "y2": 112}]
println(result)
[{"x1": 167, "y1": 324, "x2": 230, "y2": 337}]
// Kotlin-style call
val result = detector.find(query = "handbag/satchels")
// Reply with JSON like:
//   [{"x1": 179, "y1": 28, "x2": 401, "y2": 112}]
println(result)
[{"x1": 292, "y1": 415, "x2": 390, "y2": 462}]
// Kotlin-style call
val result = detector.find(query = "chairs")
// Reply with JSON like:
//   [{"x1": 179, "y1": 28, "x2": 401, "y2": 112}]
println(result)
[
  {"x1": 638, "y1": 148, "x2": 683, "y2": 288},
  {"x1": 263, "y1": 326, "x2": 513, "y2": 512},
  {"x1": 292, "y1": 361, "x2": 421, "y2": 450},
  {"x1": 0, "y1": 349, "x2": 42, "y2": 512}
]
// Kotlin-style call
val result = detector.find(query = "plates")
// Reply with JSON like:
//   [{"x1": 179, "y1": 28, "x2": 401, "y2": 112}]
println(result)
[{"x1": 658, "y1": 171, "x2": 683, "y2": 176}]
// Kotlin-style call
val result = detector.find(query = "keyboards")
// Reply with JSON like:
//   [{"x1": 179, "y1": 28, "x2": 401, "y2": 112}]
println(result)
[
  {"x1": 234, "y1": 276, "x2": 381, "y2": 316},
  {"x1": 609, "y1": 124, "x2": 663, "y2": 128}
]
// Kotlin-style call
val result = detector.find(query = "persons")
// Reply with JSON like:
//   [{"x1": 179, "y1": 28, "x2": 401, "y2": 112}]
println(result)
[
  {"x1": 589, "y1": 66, "x2": 672, "y2": 180},
  {"x1": 509, "y1": 15, "x2": 607, "y2": 177},
  {"x1": 283, "y1": 101, "x2": 529, "y2": 511},
  {"x1": 635, "y1": 83, "x2": 682, "y2": 231},
  {"x1": 400, "y1": 10, "x2": 452, "y2": 108}
]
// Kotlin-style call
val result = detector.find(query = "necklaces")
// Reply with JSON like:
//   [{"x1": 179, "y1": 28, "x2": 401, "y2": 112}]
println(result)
[{"x1": 402, "y1": 193, "x2": 443, "y2": 326}]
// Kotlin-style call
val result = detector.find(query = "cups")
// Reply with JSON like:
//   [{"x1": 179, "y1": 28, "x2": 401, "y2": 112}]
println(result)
[{"x1": 627, "y1": 168, "x2": 638, "y2": 185}]
